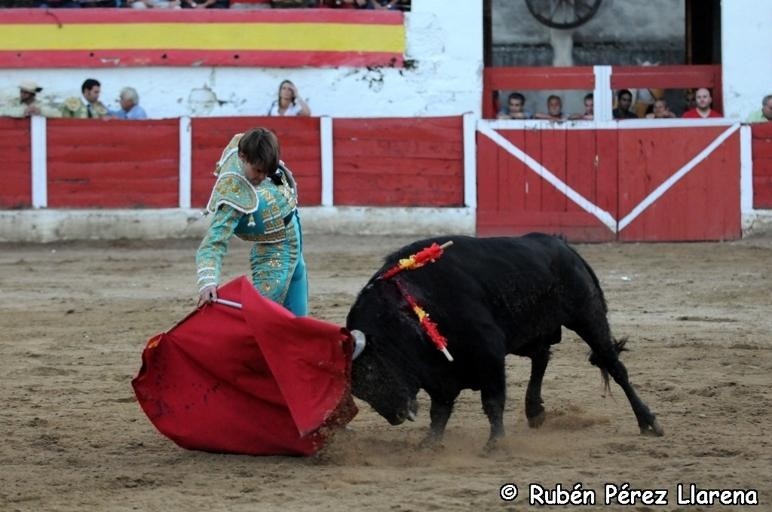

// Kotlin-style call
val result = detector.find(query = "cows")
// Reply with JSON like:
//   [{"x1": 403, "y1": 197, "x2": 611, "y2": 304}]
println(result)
[{"x1": 345, "y1": 230, "x2": 665, "y2": 458}]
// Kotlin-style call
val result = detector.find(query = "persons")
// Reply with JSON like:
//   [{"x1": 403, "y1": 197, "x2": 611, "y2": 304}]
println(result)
[
  {"x1": 267, "y1": 80, "x2": 311, "y2": 117},
  {"x1": 681, "y1": 88, "x2": 722, "y2": 118},
  {"x1": 2, "y1": 81, "x2": 61, "y2": 118},
  {"x1": 0, "y1": 0, "x2": 411, "y2": 12},
  {"x1": 195, "y1": 127, "x2": 308, "y2": 317},
  {"x1": 568, "y1": 93, "x2": 593, "y2": 120},
  {"x1": 533, "y1": 95, "x2": 581, "y2": 119},
  {"x1": 613, "y1": 89, "x2": 638, "y2": 119},
  {"x1": 646, "y1": 99, "x2": 676, "y2": 119},
  {"x1": 496, "y1": 93, "x2": 534, "y2": 119},
  {"x1": 59, "y1": 79, "x2": 110, "y2": 119},
  {"x1": 743, "y1": 95, "x2": 772, "y2": 123},
  {"x1": 108, "y1": 87, "x2": 147, "y2": 120}
]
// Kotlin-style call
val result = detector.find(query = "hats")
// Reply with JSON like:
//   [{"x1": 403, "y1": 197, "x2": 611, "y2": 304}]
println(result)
[{"x1": 18, "y1": 79, "x2": 36, "y2": 93}]
